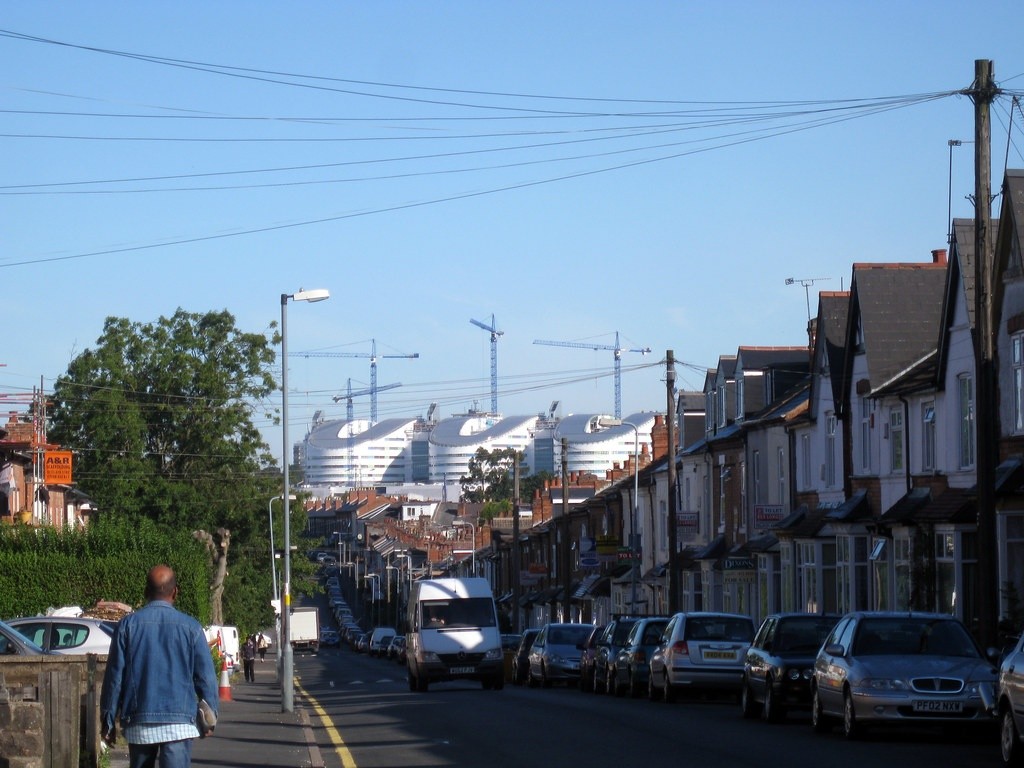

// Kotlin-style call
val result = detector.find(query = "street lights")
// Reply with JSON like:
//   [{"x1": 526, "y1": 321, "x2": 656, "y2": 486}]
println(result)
[
  {"x1": 333, "y1": 531, "x2": 357, "y2": 585},
  {"x1": 280, "y1": 288, "x2": 331, "y2": 711},
  {"x1": 269, "y1": 494, "x2": 297, "y2": 682},
  {"x1": 452, "y1": 521, "x2": 475, "y2": 578},
  {"x1": 397, "y1": 555, "x2": 412, "y2": 591},
  {"x1": 598, "y1": 418, "x2": 639, "y2": 612},
  {"x1": 386, "y1": 566, "x2": 400, "y2": 633},
  {"x1": 363, "y1": 573, "x2": 381, "y2": 628}
]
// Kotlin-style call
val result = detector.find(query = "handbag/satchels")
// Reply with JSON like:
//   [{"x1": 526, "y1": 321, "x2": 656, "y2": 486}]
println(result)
[{"x1": 197, "y1": 699, "x2": 217, "y2": 739}]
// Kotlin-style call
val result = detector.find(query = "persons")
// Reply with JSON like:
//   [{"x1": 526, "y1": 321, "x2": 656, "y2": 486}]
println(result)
[
  {"x1": 258, "y1": 635, "x2": 268, "y2": 662},
  {"x1": 100, "y1": 566, "x2": 219, "y2": 768},
  {"x1": 241, "y1": 637, "x2": 256, "y2": 681},
  {"x1": 424, "y1": 606, "x2": 448, "y2": 624}
]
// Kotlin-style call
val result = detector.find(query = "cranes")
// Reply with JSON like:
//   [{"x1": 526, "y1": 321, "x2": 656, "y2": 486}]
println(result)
[
  {"x1": 531, "y1": 331, "x2": 652, "y2": 419},
  {"x1": 470, "y1": 313, "x2": 504, "y2": 415},
  {"x1": 270, "y1": 339, "x2": 420, "y2": 422}
]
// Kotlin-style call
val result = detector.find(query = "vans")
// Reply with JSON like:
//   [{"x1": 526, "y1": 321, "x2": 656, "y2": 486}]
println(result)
[
  {"x1": 200, "y1": 625, "x2": 241, "y2": 672},
  {"x1": 405, "y1": 576, "x2": 504, "y2": 694},
  {"x1": 369, "y1": 628, "x2": 398, "y2": 656}
]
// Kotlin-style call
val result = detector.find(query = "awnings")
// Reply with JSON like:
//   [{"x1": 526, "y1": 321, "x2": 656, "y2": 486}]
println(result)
[
  {"x1": 880, "y1": 454, "x2": 1024, "y2": 520},
  {"x1": 651, "y1": 548, "x2": 698, "y2": 577},
  {"x1": 776, "y1": 490, "x2": 869, "y2": 537},
  {"x1": 692, "y1": 535, "x2": 779, "y2": 558},
  {"x1": 495, "y1": 593, "x2": 513, "y2": 602},
  {"x1": 520, "y1": 588, "x2": 563, "y2": 606},
  {"x1": 575, "y1": 564, "x2": 631, "y2": 597}
]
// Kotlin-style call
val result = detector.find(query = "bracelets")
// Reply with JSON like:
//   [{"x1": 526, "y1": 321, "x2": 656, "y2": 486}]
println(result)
[{"x1": 437, "y1": 619, "x2": 440, "y2": 622}]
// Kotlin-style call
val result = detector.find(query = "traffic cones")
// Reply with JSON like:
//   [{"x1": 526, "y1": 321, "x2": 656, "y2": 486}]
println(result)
[{"x1": 218, "y1": 662, "x2": 232, "y2": 702}]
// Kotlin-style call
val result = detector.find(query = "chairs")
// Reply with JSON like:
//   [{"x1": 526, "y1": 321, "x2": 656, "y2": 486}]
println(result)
[
  {"x1": 783, "y1": 633, "x2": 802, "y2": 649},
  {"x1": 857, "y1": 633, "x2": 883, "y2": 651},
  {"x1": 40, "y1": 627, "x2": 60, "y2": 650}
]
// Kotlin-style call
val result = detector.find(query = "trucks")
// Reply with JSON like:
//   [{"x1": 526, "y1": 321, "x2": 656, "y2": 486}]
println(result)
[{"x1": 289, "y1": 607, "x2": 320, "y2": 654}]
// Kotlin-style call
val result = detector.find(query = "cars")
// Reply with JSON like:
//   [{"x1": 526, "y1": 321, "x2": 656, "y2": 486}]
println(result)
[
  {"x1": 316, "y1": 552, "x2": 370, "y2": 651},
  {"x1": 498, "y1": 611, "x2": 843, "y2": 726},
  {"x1": 209, "y1": 647, "x2": 234, "y2": 676},
  {"x1": 809, "y1": 609, "x2": 1024, "y2": 768},
  {"x1": 1, "y1": 616, "x2": 121, "y2": 656},
  {"x1": 378, "y1": 635, "x2": 407, "y2": 666}
]
